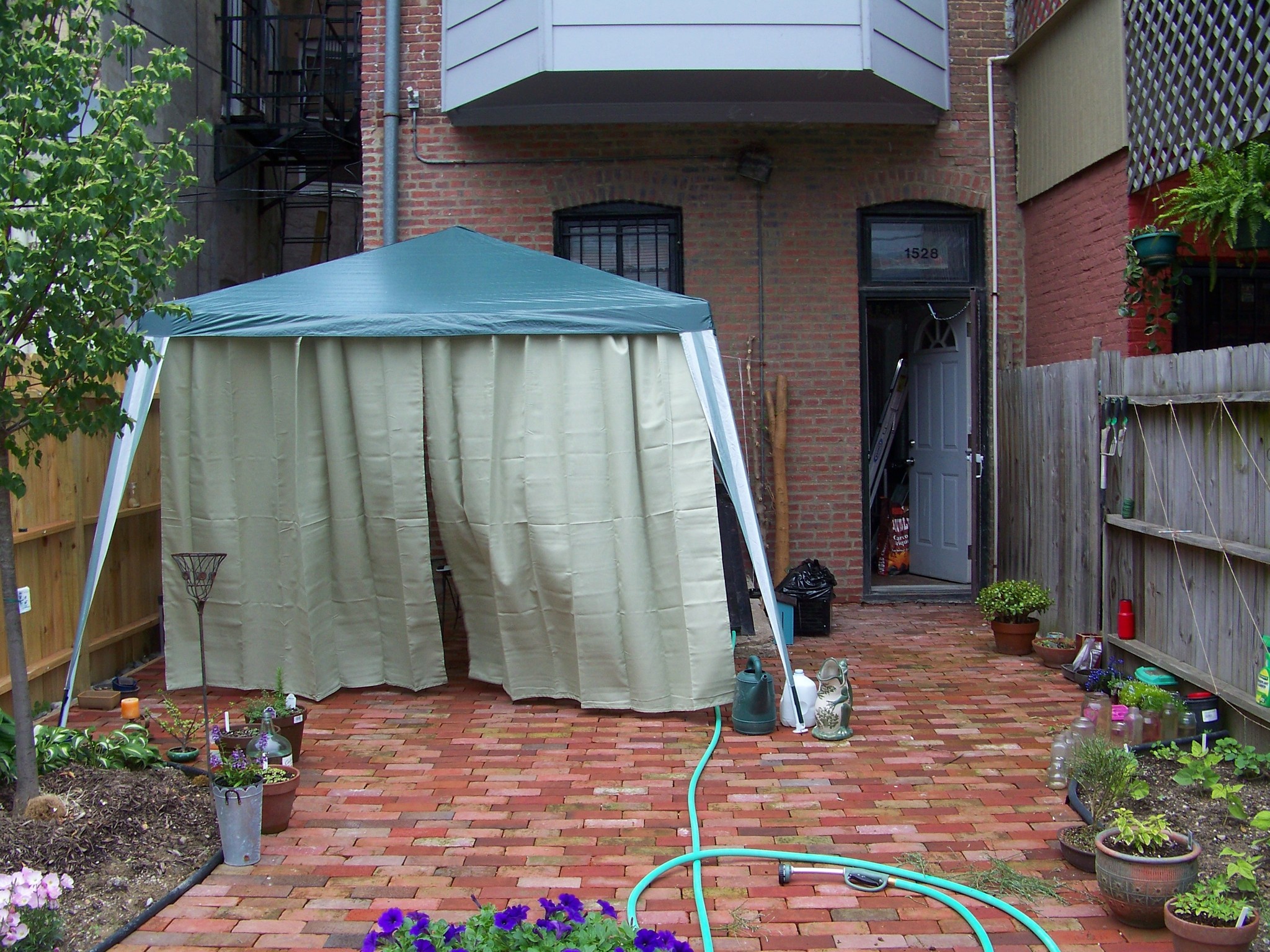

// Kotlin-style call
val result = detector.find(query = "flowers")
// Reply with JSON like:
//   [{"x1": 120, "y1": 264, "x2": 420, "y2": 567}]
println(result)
[
  {"x1": 1085, "y1": 655, "x2": 1188, "y2": 716},
  {"x1": 201, "y1": 725, "x2": 272, "y2": 784}
]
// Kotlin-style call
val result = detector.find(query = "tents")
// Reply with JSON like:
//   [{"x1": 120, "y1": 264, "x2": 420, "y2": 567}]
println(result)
[{"x1": 54, "y1": 227, "x2": 810, "y2": 735}]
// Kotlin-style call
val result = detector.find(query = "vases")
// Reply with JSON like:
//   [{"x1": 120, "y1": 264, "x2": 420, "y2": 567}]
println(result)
[{"x1": 212, "y1": 774, "x2": 264, "y2": 866}]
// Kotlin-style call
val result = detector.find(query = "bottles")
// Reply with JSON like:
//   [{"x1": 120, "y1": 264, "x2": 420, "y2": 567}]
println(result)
[
  {"x1": 1117, "y1": 599, "x2": 1134, "y2": 640},
  {"x1": 245, "y1": 711, "x2": 293, "y2": 766},
  {"x1": 779, "y1": 668, "x2": 818, "y2": 729},
  {"x1": 1046, "y1": 692, "x2": 1198, "y2": 790}
]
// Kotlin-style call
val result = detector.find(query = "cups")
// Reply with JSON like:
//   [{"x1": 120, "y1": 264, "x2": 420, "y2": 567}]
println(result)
[{"x1": 1047, "y1": 632, "x2": 1064, "y2": 638}]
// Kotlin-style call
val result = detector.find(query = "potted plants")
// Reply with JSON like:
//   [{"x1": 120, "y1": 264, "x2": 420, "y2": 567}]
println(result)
[
  {"x1": 215, "y1": 724, "x2": 281, "y2": 759},
  {"x1": 1118, "y1": 230, "x2": 1197, "y2": 354},
  {"x1": 254, "y1": 763, "x2": 300, "y2": 835},
  {"x1": 139, "y1": 692, "x2": 237, "y2": 761},
  {"x1": 1031, "y1": 637, "x2": 1076, "y2": 669},
  {"x1": 975, "y1": 578, "x2": 1055, "y2": 655},
  {"x1": 240, "y1": 667, "x2": 307, "y2": 762},
  {"x1": 1055, "y1": 742, "x2": 1261, "y2": 952}
]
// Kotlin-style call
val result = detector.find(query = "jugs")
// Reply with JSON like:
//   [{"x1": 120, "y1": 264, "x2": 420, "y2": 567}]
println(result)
[{"x1": 731, "y1": 655, "x2": 777, "y2": 736}]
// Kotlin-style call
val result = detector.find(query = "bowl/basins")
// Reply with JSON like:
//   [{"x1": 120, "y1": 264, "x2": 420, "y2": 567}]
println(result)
[
  {"x1": 92, "y1": 683, "x2": 141, "y2": 702},
  {"x1": 1074, "y1": 669, "x2": 1102, "y2": 685},
  {"x1": 112, "y1": 677, "x2": 137, "y2": 691}
]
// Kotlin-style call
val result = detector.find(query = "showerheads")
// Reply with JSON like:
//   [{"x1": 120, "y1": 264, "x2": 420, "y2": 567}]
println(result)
[{"x1": 778, "y1": 862, "x2": 793, "y2": 884}]
